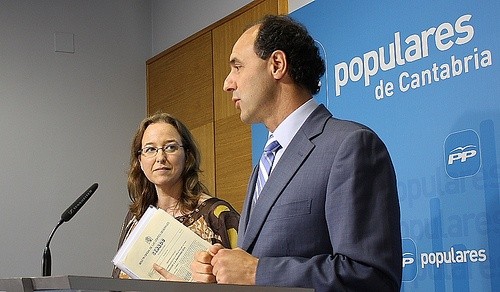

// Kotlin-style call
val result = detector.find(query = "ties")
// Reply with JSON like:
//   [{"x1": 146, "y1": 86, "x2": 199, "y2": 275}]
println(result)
[{"x1": 249, "y1": 140, "x2": 283, "y2": 212}]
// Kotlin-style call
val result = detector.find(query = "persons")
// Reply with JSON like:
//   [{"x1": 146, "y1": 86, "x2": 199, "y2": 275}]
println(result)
[
  {"x1": 190, "y1": 14, "x2": 403, "y2": 292},
  {"x1": 112, "y1": 114, "x2": 240, "y2": 279}
]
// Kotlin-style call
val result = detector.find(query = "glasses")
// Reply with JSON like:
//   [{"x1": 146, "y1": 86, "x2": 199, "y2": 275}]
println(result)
[{"x1": 138, "y1": 144, "x2": 184, "y2": 158}]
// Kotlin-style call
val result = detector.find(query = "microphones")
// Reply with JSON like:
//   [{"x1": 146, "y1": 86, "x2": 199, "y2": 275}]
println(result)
[{"x1": 43, "y1": 183, "x2": 98, "y2": 277}]
